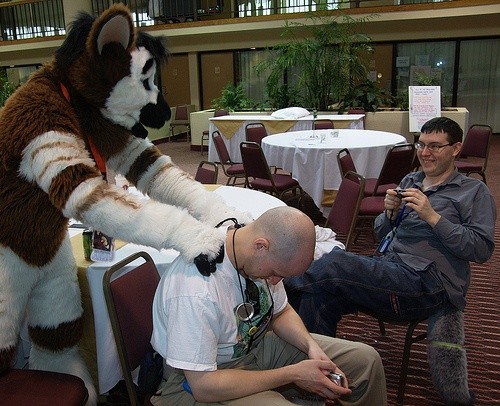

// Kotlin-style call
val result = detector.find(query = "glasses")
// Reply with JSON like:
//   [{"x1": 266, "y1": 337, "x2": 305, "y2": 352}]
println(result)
[
  {"x1": 245, "y1": 279, "x2": 260, "y2": 320},
  {"x1": 415, "y1": 141, "x2": 456, "y2": 151}
]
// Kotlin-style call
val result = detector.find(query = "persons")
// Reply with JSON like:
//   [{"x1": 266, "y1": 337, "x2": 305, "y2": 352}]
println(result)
[
  {"x1": 150, "y1": 207, "x2": 388, "y2": 406},
  {"x1": 284, "y1": 117, "x2": 497, "y2": 400}
]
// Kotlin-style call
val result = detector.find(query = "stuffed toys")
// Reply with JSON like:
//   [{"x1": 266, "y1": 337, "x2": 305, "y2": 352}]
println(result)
[{"x1": 0, "y1": 3, "x2": 254, "y2": 406}]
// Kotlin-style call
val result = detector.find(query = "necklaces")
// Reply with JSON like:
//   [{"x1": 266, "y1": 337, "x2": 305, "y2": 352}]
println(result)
[{"x1": 232, "y1": 226, "x2": 257, "y2": 336}]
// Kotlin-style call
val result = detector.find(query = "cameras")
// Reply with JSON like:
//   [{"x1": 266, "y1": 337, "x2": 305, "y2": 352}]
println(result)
[
  {"x1": 393, "y1": 189, "x2": 407, "y2": 199},
  {"x1": 326, "y1": 372, "x2": 344, "y2": 387}
]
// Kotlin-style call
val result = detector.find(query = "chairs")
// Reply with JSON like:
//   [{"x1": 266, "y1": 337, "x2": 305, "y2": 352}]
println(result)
[{"x1": 0, "y1": 104, "x2": 494, "y2": 406}]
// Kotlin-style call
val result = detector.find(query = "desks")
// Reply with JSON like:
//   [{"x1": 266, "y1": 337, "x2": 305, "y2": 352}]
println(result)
[
  {"x1": 15, "y1": 184, "x2": 289, "y2": 406},
  {"x1": 208, "y1": 115, "x2": 367, "y2": 163},
  {"x1": 261, "y1": 129, "x2": 409, "y2": 210}
]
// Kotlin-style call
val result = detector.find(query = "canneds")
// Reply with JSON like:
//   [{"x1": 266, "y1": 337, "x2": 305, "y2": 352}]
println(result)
[{"x1": 82, "y1": 226, "x2": 92, "y2": 260}]
